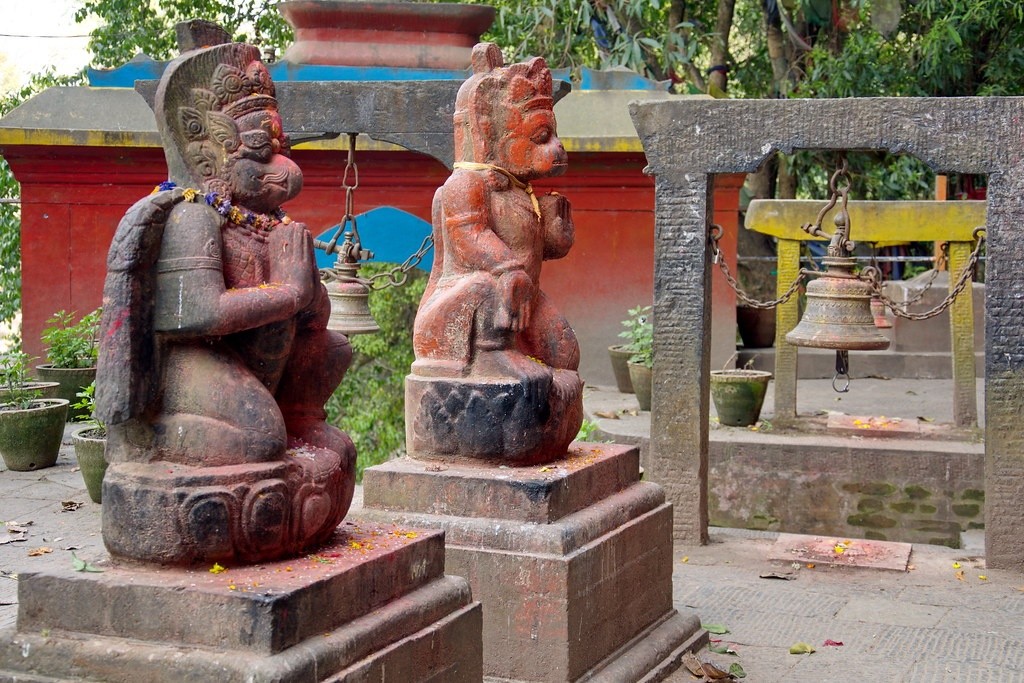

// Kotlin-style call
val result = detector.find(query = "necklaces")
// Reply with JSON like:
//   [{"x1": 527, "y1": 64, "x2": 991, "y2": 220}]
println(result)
[{"x1": 153, "y1": 183, "x2": 285, "y2": 233}]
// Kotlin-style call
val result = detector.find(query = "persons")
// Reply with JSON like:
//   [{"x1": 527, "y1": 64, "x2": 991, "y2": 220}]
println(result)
[
  {"x1": 411, "y1": 42, "x2": 581, "y2": 375},
  {"x1": 95, "y1": 20, "x2": 358, "y2": 469}
]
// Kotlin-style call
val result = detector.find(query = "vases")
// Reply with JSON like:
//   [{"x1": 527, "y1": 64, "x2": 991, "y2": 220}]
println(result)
[
  {"x1": 737, "y1": 307, "x2": 776, "y2": 349},
  {"x1": 709, "y1": 370, "x2": 772, "y2": 427}
]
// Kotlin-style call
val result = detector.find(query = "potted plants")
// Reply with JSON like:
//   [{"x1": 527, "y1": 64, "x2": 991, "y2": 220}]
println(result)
[
  {"x1": 0, "y1": 307, "x2": 108, "y2": 503},
  {"x1": 608, "y1": 304, "x2": 653, "y2": 411}
]
